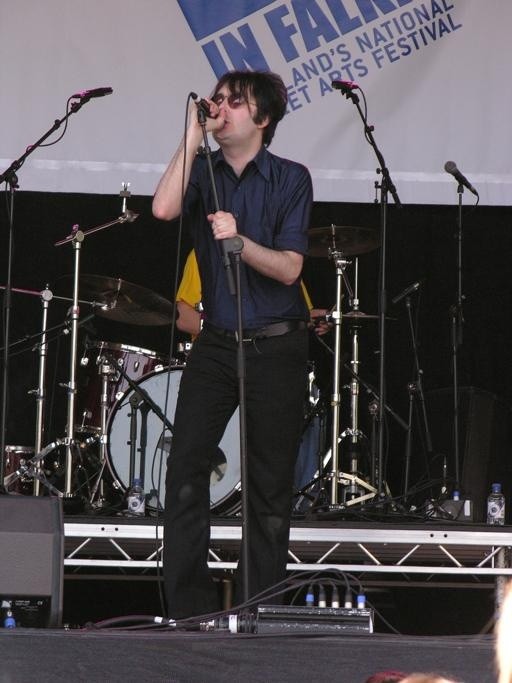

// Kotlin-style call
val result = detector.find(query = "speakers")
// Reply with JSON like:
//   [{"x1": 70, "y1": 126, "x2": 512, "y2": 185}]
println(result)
[{"x1": 0, "y1": 496, "x2": 62, "y2": 629}]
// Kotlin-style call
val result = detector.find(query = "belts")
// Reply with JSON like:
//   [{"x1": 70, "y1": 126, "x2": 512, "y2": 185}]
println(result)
[{"x1": 203, "y1": 318, "x2": 306, "y2": 343}]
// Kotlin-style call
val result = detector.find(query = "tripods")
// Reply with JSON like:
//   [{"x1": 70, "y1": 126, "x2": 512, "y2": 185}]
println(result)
[
  {"x1": 0, "y1": 104, "x2": 136, "y2": 496},
  {"x1": 294, "y1": 126, "x2": 452, "y2": 519}
]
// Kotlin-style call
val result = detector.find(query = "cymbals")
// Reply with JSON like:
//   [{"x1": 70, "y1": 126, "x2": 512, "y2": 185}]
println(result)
[
  {"x1": 60, "y1": 274, "x2": 179, "y2": 325},
  {"x1": 304, "y1": 227, "x2": 387, "y2": 258},
  {"x1": 325, "y1": 313, "x2": 396, "y2": 326}
]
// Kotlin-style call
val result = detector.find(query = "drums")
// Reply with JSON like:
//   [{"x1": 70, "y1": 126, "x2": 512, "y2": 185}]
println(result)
[
  {"x1": 104, "y1": 361, "x2": 241, "y2": 515},
  {"x1": 4, "y1": 445, "x2": 67, "y2": 498},
  {"x1": 64, "y1": 340, "x2": 180, "y2": 437}
]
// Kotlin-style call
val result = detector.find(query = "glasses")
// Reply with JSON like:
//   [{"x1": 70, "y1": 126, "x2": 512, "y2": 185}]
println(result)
[{"x1": 211, "y1": 93, "x2": 260, "y2": 108}]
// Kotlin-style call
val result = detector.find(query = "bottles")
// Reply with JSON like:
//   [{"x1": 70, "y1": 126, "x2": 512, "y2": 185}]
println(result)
[
  {"x1": 486, "y1": 483, "x2": 506, "y2": 526},
  {"x1": 126, "y1": 479, "x2": 147, "y2": 517}
]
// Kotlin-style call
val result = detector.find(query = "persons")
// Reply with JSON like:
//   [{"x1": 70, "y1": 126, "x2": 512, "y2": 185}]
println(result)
[
  {"x1": 176, "y1": 242, "x2": 342, "y2": 518},
  {"x1": 151, "y1": 71, "x2": 314, "y2": 620}
]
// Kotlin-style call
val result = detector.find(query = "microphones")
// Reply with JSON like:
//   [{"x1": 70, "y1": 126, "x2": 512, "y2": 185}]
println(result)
[
  {"x1": 191, "y1": 94, "x2": 218, "y2": 119},
  {"x1": 71, "y1": 86, "x2": 113, "y2": 100},
  {"x1": 332, "y1": 81, "x2": 358, "y2": 92},
  {"x1": 443, "y1": 160, "x2": 477, "y2": 197}
]
